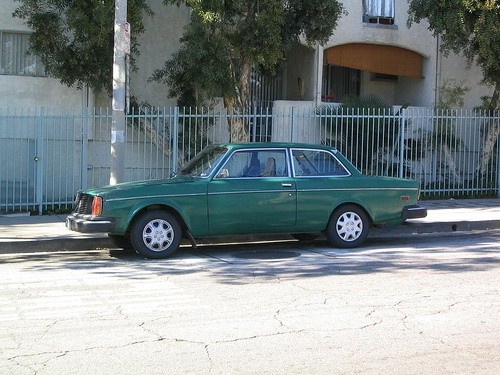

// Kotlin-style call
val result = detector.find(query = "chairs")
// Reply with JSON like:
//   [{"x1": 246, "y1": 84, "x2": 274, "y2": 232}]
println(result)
[{"x1": 242, "y1": 157, "x2": 277, "y2": 177}]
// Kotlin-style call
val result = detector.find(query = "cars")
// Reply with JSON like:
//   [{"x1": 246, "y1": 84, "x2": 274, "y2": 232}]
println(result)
[{"x1": 65, "y1": 142, "x2": 428, "y2": 260}]
